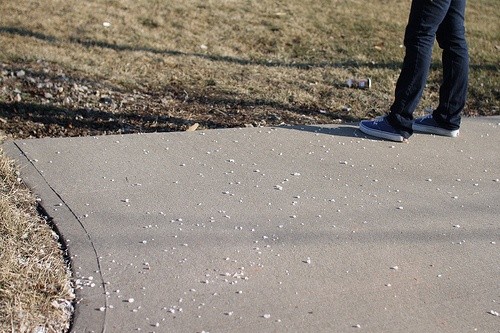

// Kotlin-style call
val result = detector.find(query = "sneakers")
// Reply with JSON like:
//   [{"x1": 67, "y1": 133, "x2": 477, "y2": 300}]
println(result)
[
  {"x1": 359, "y1": 115, "x2": 402, "y2": 141},
  {"x1": 410, "y1": 114, "x2": 459, "y2": 136}
]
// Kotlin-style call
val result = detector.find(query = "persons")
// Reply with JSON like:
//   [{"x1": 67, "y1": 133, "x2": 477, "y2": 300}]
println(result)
[{"x1": 356, "y1": 0, "x2": 470, "y2": 142}]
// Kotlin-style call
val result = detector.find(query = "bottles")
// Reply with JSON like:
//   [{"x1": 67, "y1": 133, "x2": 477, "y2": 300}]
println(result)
[{"x1": 346, "y1": 79, "x2": 372, "y2": 89}]
